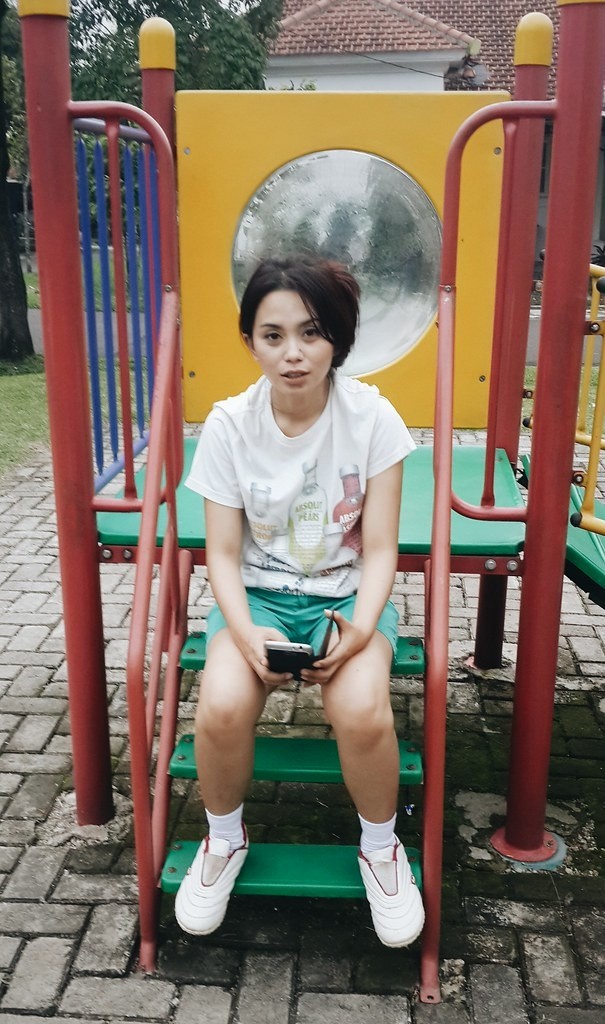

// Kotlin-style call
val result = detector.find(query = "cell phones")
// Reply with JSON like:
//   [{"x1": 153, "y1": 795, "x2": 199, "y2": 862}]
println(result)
[{"x1": 263, "y1": 641, "x2": 314, "y2": 681}]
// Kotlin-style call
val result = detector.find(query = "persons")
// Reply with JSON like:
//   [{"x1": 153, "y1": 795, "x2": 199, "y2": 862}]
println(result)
[{"x1": 174, "y1": 250, "x2": 426, "y2": 949}]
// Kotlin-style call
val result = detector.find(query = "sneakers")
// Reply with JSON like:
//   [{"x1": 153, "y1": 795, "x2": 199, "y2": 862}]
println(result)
[
  {"x1": 175, "y1": 820, "x2": 249, "y2": 935},
  {"x1": 357, "y1": 833, "x2": 426, "y2": 948}
]
